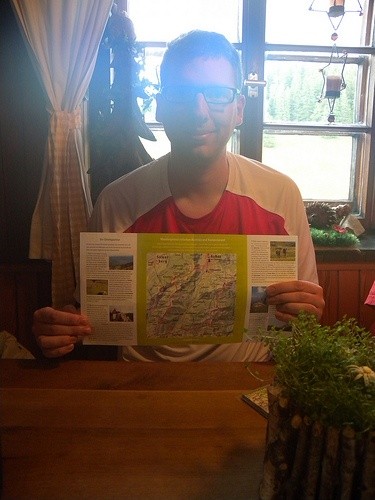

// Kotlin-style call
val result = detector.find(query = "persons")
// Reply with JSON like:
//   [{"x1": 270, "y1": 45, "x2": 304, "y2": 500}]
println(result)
[{"x1": 33, "y1": 30, "x2": 326, "y2": 364}]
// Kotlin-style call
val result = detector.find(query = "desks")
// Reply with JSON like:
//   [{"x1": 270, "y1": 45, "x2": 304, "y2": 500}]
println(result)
[{"x1": 0, "y1": 358, "x2": 282, "y2": 500}]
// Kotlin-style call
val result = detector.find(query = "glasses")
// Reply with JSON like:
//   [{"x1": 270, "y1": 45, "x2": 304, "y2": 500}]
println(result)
[{"x1": 162, "y1": 86, "x2": 241, "y2": 105}]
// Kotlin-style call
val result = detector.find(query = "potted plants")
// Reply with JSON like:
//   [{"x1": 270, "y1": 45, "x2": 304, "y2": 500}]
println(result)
[{"x1": 241, "y1": 308, "x2": 375, "y2": 500}]
